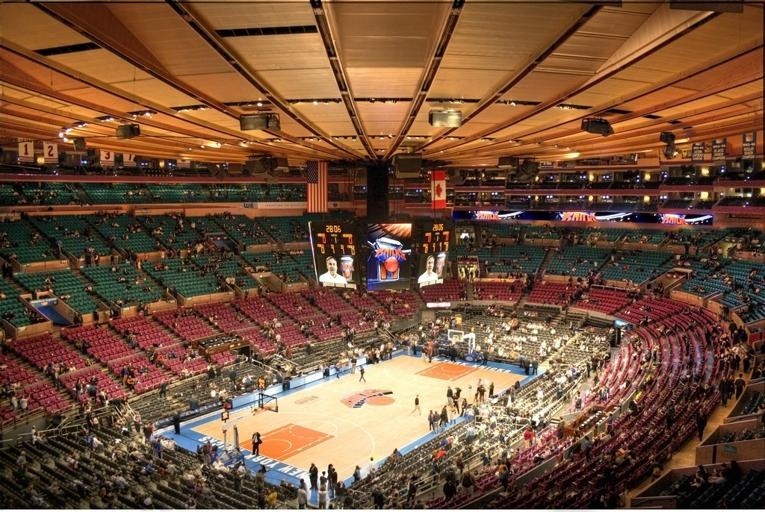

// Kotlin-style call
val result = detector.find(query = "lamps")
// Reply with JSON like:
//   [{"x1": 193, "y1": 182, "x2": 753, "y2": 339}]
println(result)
[
  {"x1": 663, "y1": 144, "x2": 676, "y2": 159},
  {"x1": 428, "y1": 108, "x2": 463, "y2": 128},
  {"x1": 659, "y1": 132, "x2": 675, "y2": 144},
  {"x1": 116, "y1": 123, "x2": 141, "y2": 139},
  {"x1": 245, "y1": 152, "x2": 272, "y2": 176},
  {"x1": 227, "y1": 163, "x2": 244, "y2": 174},
  {"x1": 240, "y1": 111, "x2": 282, "y2": 132},
  {"x1": 581, "y1": 116, "x2": 614, "y2": 136}
]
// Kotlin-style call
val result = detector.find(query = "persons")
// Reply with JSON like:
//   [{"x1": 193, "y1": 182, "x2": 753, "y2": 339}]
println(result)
[{"x1": 1, "y1": 174, "x2": 765, "y2": 510}]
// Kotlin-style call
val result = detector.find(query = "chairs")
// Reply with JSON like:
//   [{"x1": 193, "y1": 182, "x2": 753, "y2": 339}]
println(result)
[{"x1": 0, "y1": 176, "x2": 760, "y2": 509}]
